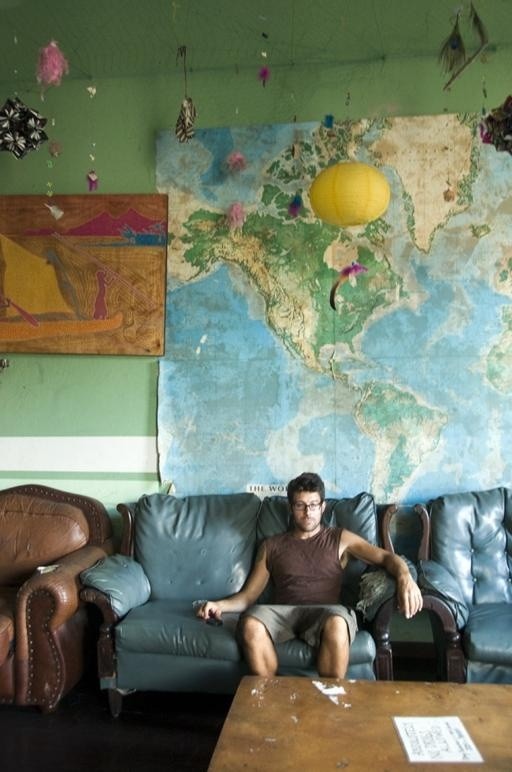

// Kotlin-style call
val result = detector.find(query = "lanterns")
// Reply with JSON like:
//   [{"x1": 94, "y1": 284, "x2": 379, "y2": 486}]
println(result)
[{"x1": 309, "y1": 164, "x2": 391, "y2": 230}]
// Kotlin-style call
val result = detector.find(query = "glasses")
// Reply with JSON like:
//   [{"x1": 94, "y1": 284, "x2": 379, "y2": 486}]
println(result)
[{"x1": 292, "y1": 500, "x2": 323, "y2": 510}]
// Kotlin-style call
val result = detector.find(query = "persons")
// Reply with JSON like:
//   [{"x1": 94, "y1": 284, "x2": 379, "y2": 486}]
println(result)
[{"x1": 196, "y1": 473, "x2": 423, "y2": 679}]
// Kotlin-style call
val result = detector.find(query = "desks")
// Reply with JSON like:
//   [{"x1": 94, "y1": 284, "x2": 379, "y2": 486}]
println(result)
[{"x1": 210, "y1": 674, "x2": 511, "y2": 769}]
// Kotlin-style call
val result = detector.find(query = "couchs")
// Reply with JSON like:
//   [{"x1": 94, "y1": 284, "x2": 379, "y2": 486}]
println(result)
[
  {"x1": 415, "y1": 487, "x2": 512, "y2": 686},
  {"x1": 0, "y1": 484, "x2": 117, "y2": 712},
  {"x1": 81, "y1": 493, "x2": 417, "y2": 718}
]
